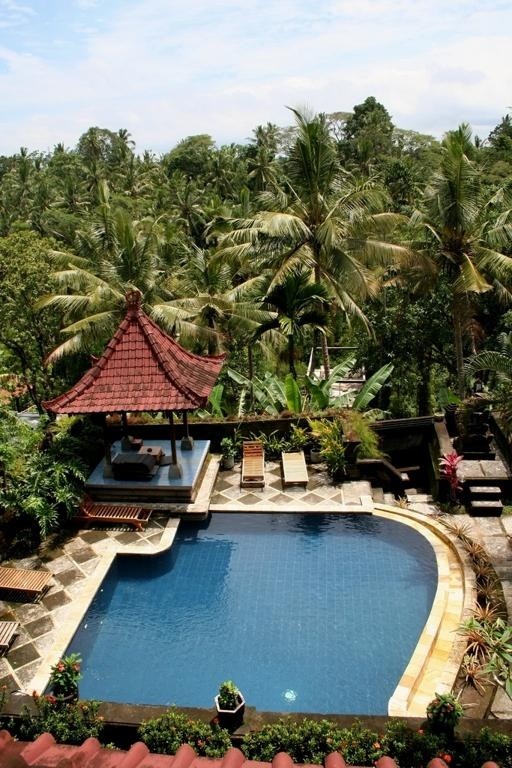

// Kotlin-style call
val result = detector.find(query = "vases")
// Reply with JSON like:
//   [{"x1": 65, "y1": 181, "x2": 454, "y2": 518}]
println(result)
[
  {"x1": 426, "y1": 707, "x2": 463, "y2": 735},
  {"x1": 49, "y1": 687, "x2": 79, "y2": 705}
]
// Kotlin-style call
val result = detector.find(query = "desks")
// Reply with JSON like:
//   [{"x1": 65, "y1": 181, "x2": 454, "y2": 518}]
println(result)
[{"x1": 139, "y1": 446, "x2": 162, "y2": 461}]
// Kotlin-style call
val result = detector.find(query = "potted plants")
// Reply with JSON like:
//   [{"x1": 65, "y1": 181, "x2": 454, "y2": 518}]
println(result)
[
  {"x1": 220, "y1": 437, "x2": 237, "y2": 469},
  {"x1": 310, "y1": 429, "x2": 325, "y2": 463},
  {"x1": 214, "y1": 680, "x2": 245, "y2": 726}
]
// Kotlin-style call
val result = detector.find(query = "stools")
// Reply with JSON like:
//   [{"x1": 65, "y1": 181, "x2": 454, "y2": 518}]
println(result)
[{"x1": 131, "y1": 439, "x2": 143, "y2": 450}]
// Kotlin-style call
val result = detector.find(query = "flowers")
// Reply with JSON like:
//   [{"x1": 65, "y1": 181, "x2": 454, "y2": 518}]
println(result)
[
  {"x1": 426, "y1": 692, "x2": 465, "y2": 728},
  {"x1": 47, "y1": 652, "x2": 84, "y2": 693}
]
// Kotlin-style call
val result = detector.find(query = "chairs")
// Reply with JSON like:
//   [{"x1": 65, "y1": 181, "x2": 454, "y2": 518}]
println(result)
[
  {"x1": 0, "y1": 621, "x2": 21, "y2": 658},
  {"x1": 241, "y1": 441, "x2": 265, "y2": 492},
  {"x1": 282, "y1": 450, "x2": 309, "y2": 492},
  {"x1": 79, "y1": 494, "x2": 154, "y2": 531},
  {"x1": 0, "y1": 567, "x2": 55, "y2": 602}
]
